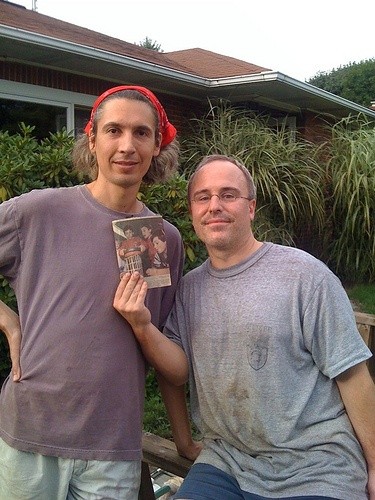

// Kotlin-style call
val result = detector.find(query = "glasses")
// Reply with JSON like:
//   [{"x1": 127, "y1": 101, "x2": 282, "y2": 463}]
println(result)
[{"x1": 191, "y1": 191, "x2": 251, "y2": 204}]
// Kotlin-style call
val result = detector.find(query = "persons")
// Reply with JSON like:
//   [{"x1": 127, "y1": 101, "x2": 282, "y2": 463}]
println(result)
[
  {"x1": 119, "y1": 223, "x2": 170, "y2": 277},
  {"x1": 0, "y1": 86, "x2": 201, "y2": 500},
  {"x1": 113, "y1": 154, "x2": 375, "y2": 500}
]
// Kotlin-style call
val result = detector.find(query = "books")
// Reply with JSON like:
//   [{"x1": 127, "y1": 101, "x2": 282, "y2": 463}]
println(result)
[{"x1": 112, "y1": 215, "x2": 171, "y2": 289}]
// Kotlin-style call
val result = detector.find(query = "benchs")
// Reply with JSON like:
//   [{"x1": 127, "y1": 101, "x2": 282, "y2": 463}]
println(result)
[{"x1": 137, "y1": 312, "x2": 375, "y2": 500}]
